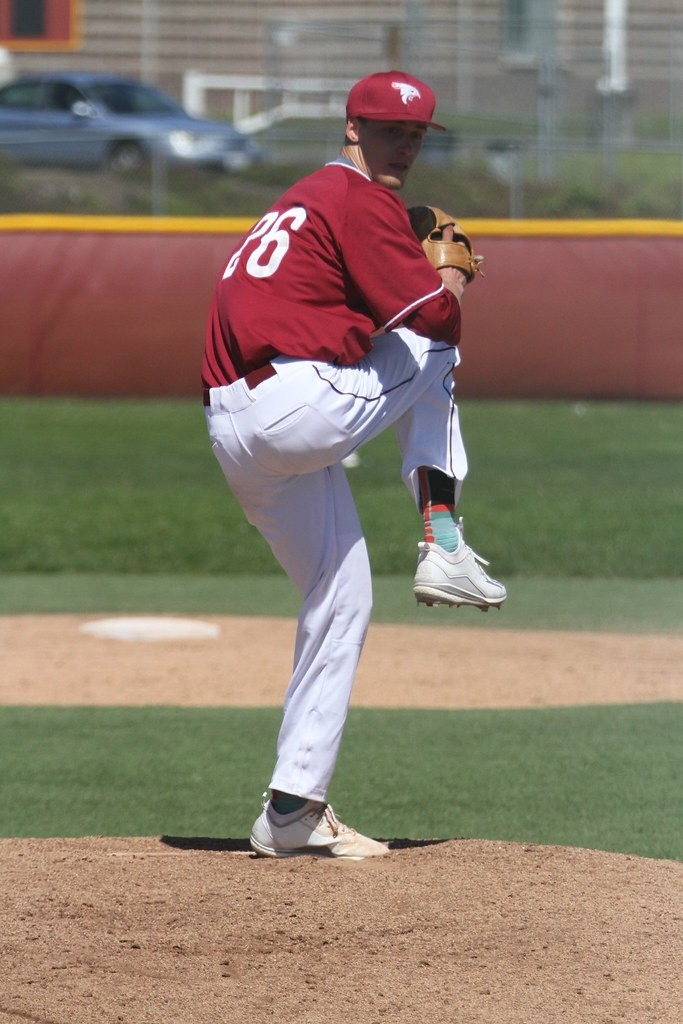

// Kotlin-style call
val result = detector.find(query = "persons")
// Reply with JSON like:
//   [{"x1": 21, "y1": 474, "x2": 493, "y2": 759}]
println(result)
[{"x1": 200, "y1": 70, "x2": 506, "y2": 859}]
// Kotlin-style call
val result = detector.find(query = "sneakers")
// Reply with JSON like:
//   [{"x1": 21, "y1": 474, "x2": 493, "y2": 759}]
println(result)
[
  {"x1": 249, "y1": 792, "x2": 391, "y2": 862},
  {"x1": 412, "y1": 517, "x2": 507, "y2": 613}
]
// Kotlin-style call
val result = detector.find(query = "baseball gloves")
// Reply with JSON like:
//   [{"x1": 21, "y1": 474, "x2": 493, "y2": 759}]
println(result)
[{"x1": 408, "y1": 205, "x2": 483, "y2": 284}]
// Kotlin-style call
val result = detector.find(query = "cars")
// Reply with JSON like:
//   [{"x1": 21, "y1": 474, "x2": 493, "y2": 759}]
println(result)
[{"x1": 0, "y1": 71, "x2": 267, "y2": 183}]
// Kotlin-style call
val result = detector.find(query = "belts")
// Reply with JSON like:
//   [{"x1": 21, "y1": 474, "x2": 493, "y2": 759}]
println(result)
[{"x1": 201, "y1": 361, "x2": 276, "y2": 406}]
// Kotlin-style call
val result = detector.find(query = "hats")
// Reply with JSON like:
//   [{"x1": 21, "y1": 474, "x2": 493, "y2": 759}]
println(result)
[{"x1": 345, "y1": 70, "x2": 450, "y2": 132}]
